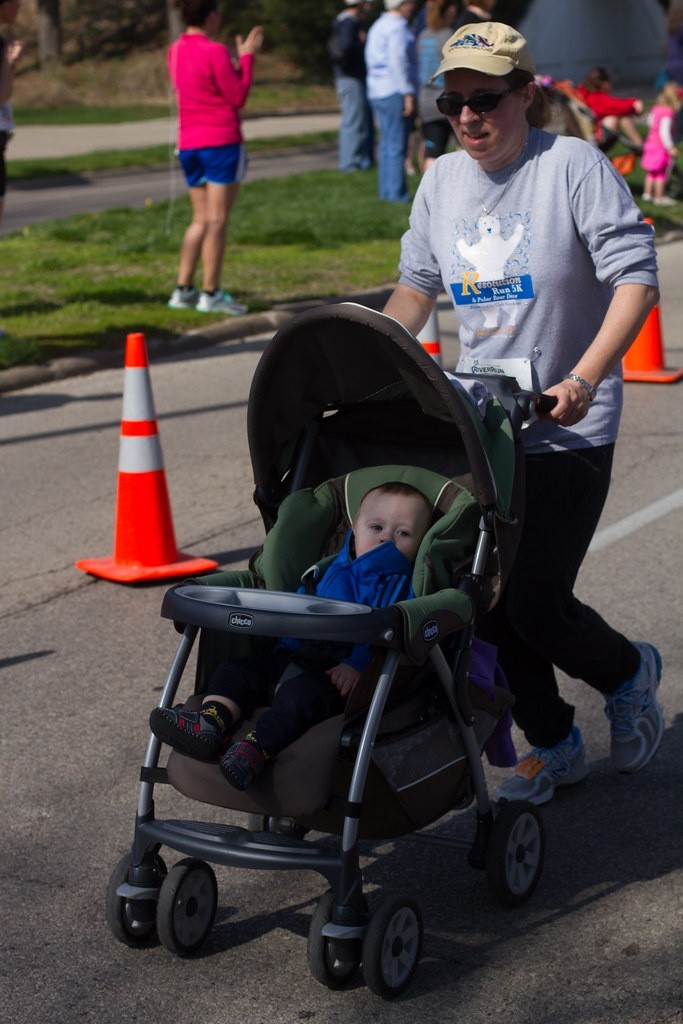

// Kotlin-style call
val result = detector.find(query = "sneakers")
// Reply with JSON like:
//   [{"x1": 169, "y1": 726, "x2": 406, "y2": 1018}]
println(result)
[
  {"x1": 606, "y1": 641, "x2": 665, "y2": 773},
  {"x1": 220, "y1": 730, "x2": 270, "y2": 791},
  {"x1": 149, "y1": 706, "x2": 231, "y2": 764},
  {"x1": 492, "y1": 726, "x2": 589, "y2": 805}
]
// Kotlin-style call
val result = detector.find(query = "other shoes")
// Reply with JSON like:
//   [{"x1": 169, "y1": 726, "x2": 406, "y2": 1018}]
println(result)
[
  {"x1": 168, "y1": 287, "x2": 200, "y2": 308},
  {"x1": 197, "y1": 291, "x2": 248, "y2": 315},
  {"x1": 643, "y1": 194, "x2": 676, "y2": 207}
]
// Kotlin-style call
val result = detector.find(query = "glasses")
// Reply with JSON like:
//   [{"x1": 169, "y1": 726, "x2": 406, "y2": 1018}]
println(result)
[{"x1": 436, "y1": 89, "x2": 515, "y2": 116}]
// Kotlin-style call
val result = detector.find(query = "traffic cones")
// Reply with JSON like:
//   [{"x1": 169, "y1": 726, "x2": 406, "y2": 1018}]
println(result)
[
  {"x1": 415, "y1": 300, "x2": 444, "y2": 369},
  {"x1": 622, "y1": 217, "x2": 683, "y2": 383},
  {"x1": 75, "y1": 333, "x2": 219, "y2": 583}
]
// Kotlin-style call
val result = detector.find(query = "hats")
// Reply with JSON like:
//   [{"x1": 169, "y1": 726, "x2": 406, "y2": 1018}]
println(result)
[{"x1": 428, "y1": 22, "x2": 527, "y2": 85}]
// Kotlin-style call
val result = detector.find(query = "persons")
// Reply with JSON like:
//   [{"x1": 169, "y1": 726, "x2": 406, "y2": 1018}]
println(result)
[
  {"x1": 0, "y1": 0, "x2": 23, "y2": 221},
  {"x1": 454, "y1": 0, "x2": 495, "y2": 29},
  {"x1": 416, "y1": 0, "x2": 453, "y2": 174},
  {"x1": 329, "y1": 0, "x2": 373, "y2": 171},
  {"x1": 641, "y1": 81, "x2": 683, "y2": 205},
  {"x1": 166, "y1": 0, "x2": 263, "y2": 318},
  {"x1": 362, "y1": 0, "x2": 417, "y2": 204},
  {"x1": 379, "y1": 21, "x2": 663, "y2": 800},
  {"x1": 149, "y1": 482, "x2": 434, "y2": 786},
  {"x1": 576, "y1": 68, "x2": 644, "y2": 155},
  {"x1": 665, "y1": 0, "x2": 683, "y2": 87}
]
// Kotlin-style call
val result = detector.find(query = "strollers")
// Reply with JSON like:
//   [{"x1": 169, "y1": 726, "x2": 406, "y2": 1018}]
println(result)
[
  {"x1": 536, "y1": 80, "x2": 683, "y2": 200},
  {"x1": 106, "y1": 300, "x2": 559, "y2": 1000}
]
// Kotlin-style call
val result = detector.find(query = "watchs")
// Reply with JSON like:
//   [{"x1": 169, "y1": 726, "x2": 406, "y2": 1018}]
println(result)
[{"x1": 563, "y1": 374, "x2": 597, "y2": 402}]
